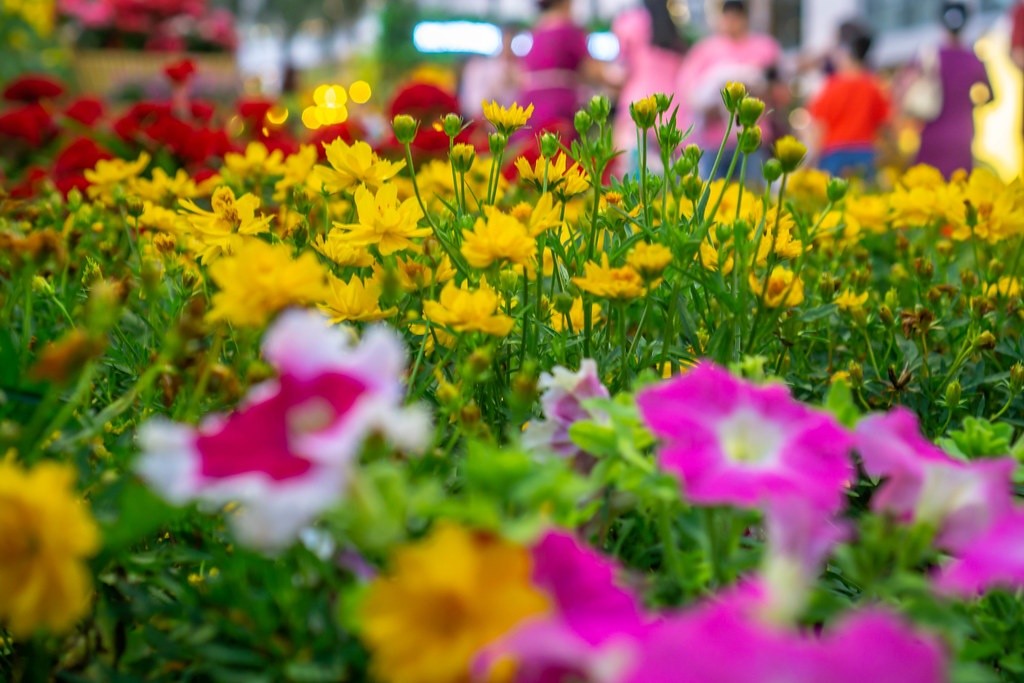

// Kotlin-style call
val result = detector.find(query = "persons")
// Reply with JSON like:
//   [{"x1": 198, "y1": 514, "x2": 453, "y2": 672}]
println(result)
[
  {"x1": 463, "y1": 21, "x2": 533, "y2": 145},
  {"x1": 507, "y1": 1, "x2": 630, "y2": 187},
  {"x1": 597, "y1": 1, "x2": 691, "y2": 195},
  {"x1": 907, "y1": 1, "x2": 996, "y2": 190},
  {"x1": 1008, "y1": 1, "x2": 1024, "y2": 146},
  {"x1": 232, "y1": 0, "x2": 369, "y2": 101},
  {"x1": 670, "y1": 1, "x2": 792, "y2": 216},
  {"x1": 803, "y1": 16, "x2": 891, "y2": 184}
]
